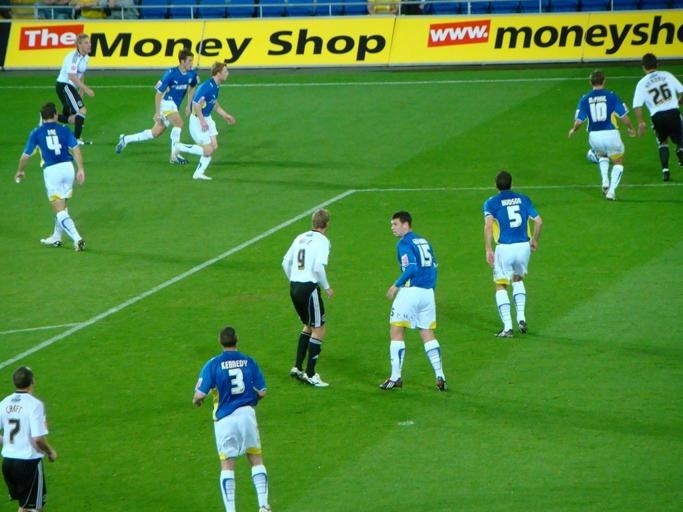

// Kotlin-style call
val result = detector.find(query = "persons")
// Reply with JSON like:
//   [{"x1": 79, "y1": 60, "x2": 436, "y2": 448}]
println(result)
[
  {"x1": 13, "y1": 102, "x2": 89, "y2": 254},
  {"x1": 0, "y1": 362, "x2": 58, "y2": 511},
  {"x1": 170, "y1": 61, "x2": 236, "y2": 182},
  {"x1": 569, "y1": 71, "x2": 637, "y2": 202},
  {"x1": 482, "y1": 172, "x2": 544, "y2": 340},
  {"x1": 631, "y1": 51, "x2": 683, "y2": 183},
  {"x1": 279, "y1": 206, "x2": 338, "y2": 389},
  {"x1": 0, "y1": 0, "x2": 139, "y2": 21},
  {"x1": 400, "y1": 0, "x2": 427, "y2": 16},
  {"x1": 39, "y1": 33, "x2": 95, "y2": 146},
  {"x1": 188, "y1": 325, "x2": 272, "y2": 511},
  {"x1": 379, "y1": 211, "x2": 451, "y2": 392},
  {"x1": 367, "y1": 0, "x2": 401, "y2": 15},
  {"x1": 113, "y1": 47, "x2": 201, "y2": 166}
]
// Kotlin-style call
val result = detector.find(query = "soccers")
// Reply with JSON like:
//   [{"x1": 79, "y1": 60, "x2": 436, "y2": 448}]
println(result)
[{"x1": 587, "y1": 149, "x2": 598, "y2": 164}]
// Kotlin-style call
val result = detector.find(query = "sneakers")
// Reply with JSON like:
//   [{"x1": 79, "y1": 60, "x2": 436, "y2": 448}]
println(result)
[
  {"x1": 73, "y1": 237, "x2": 85, "y2": 252},
  {"x1": 41, "y1": 237, "x2": 63, "y2": 248},
  {"x1": 518, "y1": 321, "x2": 527, "y2": 333},
  {"x1": 115, "y1": 134, "x2": 127, "y2": 153},
  {"x1": 169, "y1": 142, "x2": 188, "y2": 164},
  {"x1": 290, "y1": 366, "x2": 329, "y2": 387},
  {"x1": 663, "y1": 170, "x2": 670, "y2": 180},
  {"x1": 435, "y1": 376, "x2": 447, "y2": 391},
  {"x1": 259, "y1": 505, "x2": 272, "y2": 512},
  {"x1": 75, "y1": 138, "x2": 92, "y2": 145},
  {"x1": 193, "y1": 172, "x2": 213, "y2": 180},
  {"x1": 603, "y1": 184, "x2": 616, "y2": 201},
  {"x1": 495, "y1": 328, "x2": 513, "y2": 338},
  {"x1": 380, "y1": 378, "x2": 402, "y2": 389}
]
